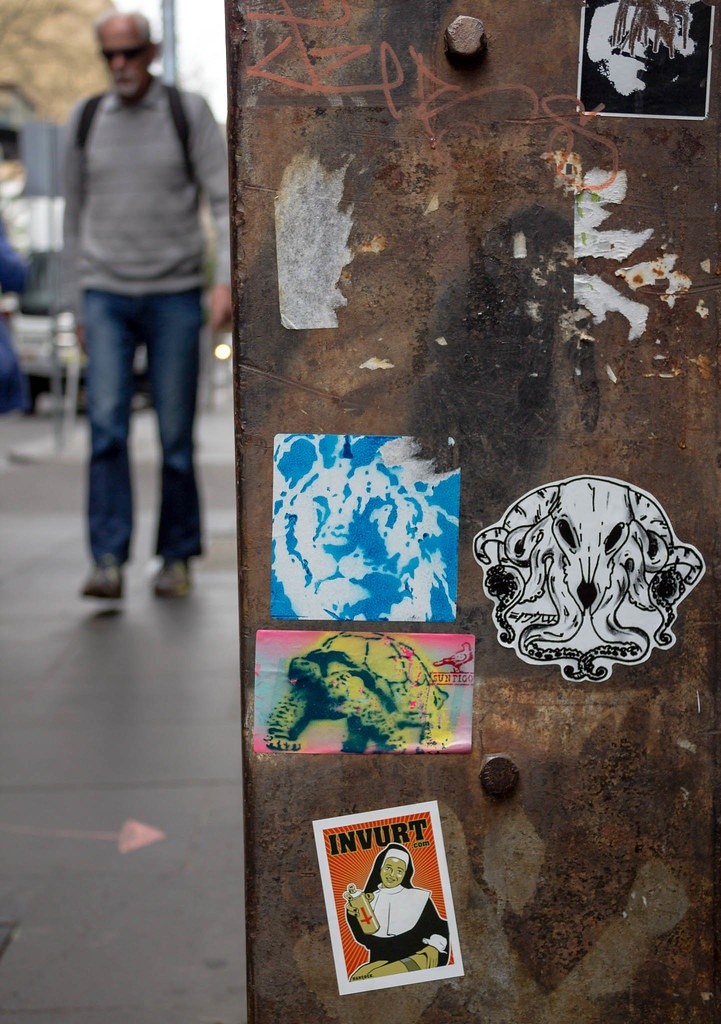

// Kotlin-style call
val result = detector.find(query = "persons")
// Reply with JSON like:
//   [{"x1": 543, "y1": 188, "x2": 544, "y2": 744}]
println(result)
[
  {"x1": 1, "y1": 211, "x2": 37, "y2": 420},
  {"x1": 64, "y1": 9, "x2": 229, "y2": 601}
]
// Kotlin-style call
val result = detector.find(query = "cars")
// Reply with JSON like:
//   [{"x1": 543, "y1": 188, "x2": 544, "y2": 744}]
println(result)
[{"x1": 12, "y1": 190, "x2": 158, "y2": 418}]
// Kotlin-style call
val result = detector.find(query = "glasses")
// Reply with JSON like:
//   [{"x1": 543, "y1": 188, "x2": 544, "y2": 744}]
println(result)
[{"x1": 102, "y1": 40, "x2": 150, "y2": 61}]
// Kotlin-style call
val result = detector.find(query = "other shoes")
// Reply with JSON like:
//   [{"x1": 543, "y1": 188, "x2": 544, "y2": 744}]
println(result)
[
  {"x1": 84, "y1": 564, "x2": 120, "y2": 598},
  {"x1": 157, "y1": 555, "x2": 191, "y2": 597}
]
